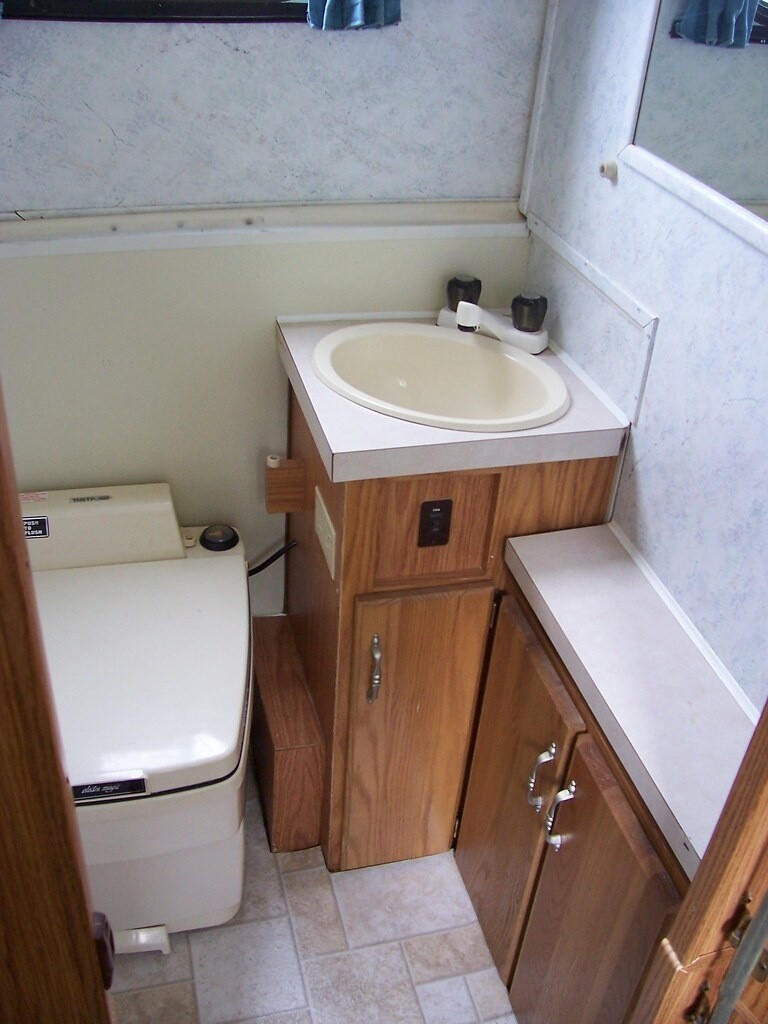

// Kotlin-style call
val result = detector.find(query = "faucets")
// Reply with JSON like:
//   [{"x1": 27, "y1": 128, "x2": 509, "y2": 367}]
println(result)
[{"x1": 455, "y1": 292, "x2": 548, "y2": 355}]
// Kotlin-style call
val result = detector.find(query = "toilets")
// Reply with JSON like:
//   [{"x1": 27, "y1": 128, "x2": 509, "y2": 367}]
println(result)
[{"x1": 18, "y1": 482, "x2": 253, "y2": 955}]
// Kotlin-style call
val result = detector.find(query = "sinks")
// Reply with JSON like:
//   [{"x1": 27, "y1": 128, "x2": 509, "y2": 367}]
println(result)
[{"x1": 310, "y1": 321, "x2": 572, "y2": 433}]
